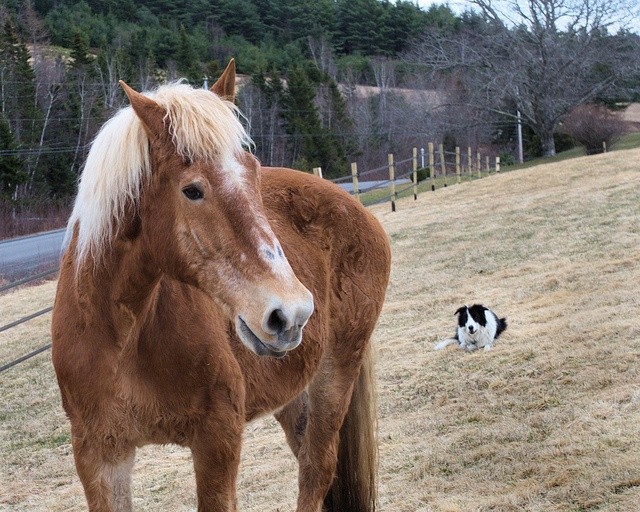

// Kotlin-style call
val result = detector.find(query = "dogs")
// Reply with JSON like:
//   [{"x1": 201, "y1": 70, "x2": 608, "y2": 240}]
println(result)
[{"x1": 433, "y1": 304, "x2": 510, "y2": 354}]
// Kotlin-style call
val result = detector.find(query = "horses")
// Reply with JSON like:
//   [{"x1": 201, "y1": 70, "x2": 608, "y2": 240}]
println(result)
[{"x1": 51, "y1": 57, "x2": 391, "y2": 512}]
